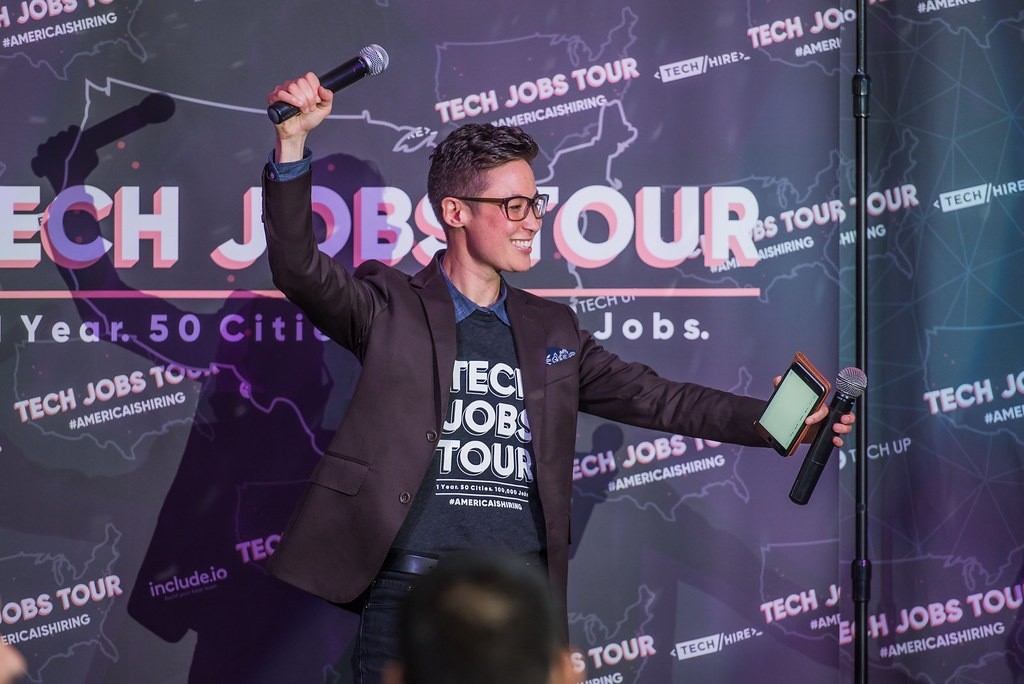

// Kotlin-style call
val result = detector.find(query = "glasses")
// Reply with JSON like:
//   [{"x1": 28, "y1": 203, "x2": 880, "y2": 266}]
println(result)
[{"x1": 447, "y1": 192, "x2": 549, "y2": 222}]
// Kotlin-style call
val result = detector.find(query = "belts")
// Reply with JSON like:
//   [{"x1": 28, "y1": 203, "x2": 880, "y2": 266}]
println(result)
[{"x1": 383, "y1": 555, "x2": 440, "y2": 577}]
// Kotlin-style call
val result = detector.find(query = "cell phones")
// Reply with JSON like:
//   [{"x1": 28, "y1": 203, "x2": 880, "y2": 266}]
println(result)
[{"x1": 752, "y1": 350, "x2": 832, "y2": 458}]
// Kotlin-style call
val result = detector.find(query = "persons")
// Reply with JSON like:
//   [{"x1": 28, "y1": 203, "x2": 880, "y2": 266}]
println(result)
[{"x1": 260, "y1": 73, "x2": 856, "y2": 684}]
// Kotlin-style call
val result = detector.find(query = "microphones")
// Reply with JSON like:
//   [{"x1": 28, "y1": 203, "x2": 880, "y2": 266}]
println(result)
[
  {"x1": 266, "y1": 44, "x2": 390, "y2": 126},
  {"x1": 788, "y1": 365, "x2": 870, "y2": 506}
]
